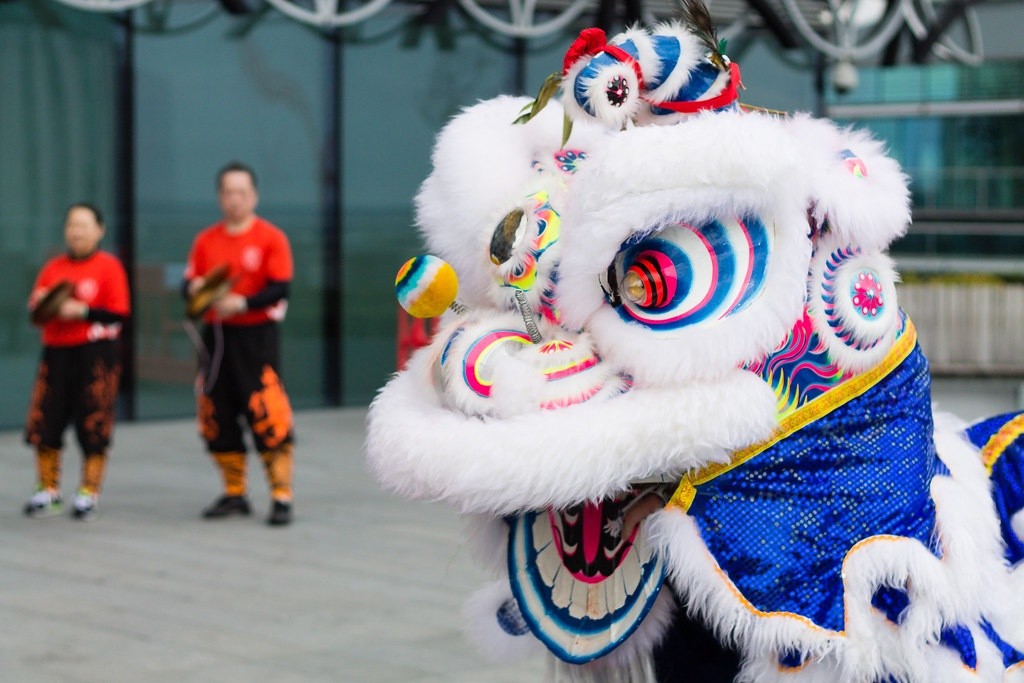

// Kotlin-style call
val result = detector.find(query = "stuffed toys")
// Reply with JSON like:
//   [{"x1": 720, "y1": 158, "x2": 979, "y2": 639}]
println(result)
[{"x1": 367, "y1": 0, "x2": 1024, "y2": 682}]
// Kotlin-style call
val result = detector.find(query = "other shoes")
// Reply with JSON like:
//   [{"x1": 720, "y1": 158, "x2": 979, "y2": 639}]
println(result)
[
  {"x1": 269, "y1": 500, "x2": 289, "y2": 524},
  {"x1": 25, "y1": 492, "x2": 62, "y2": 516},
  {"x1": 73, "y1": 492, "x2": 97, "y2": 517},
  {"x1": 204, "y1": 497, "x2": 249, "y2": 519}
]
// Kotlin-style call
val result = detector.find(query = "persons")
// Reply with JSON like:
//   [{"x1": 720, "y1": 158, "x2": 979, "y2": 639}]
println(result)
[
  {"x1": 25, "y1": 202, "x2": 131, "y2": 520},
  {"x1": 182, "y1": 164, "x2": 296, "y2": 526}
]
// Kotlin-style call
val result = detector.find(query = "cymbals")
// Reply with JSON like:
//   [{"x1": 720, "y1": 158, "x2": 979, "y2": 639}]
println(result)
[
  {"x1": 185, "y1": 264, "x2": 240, "y2": 322},
  {"x1": 30, "y1": 279, "x2": 80, "y2": 325}
]
